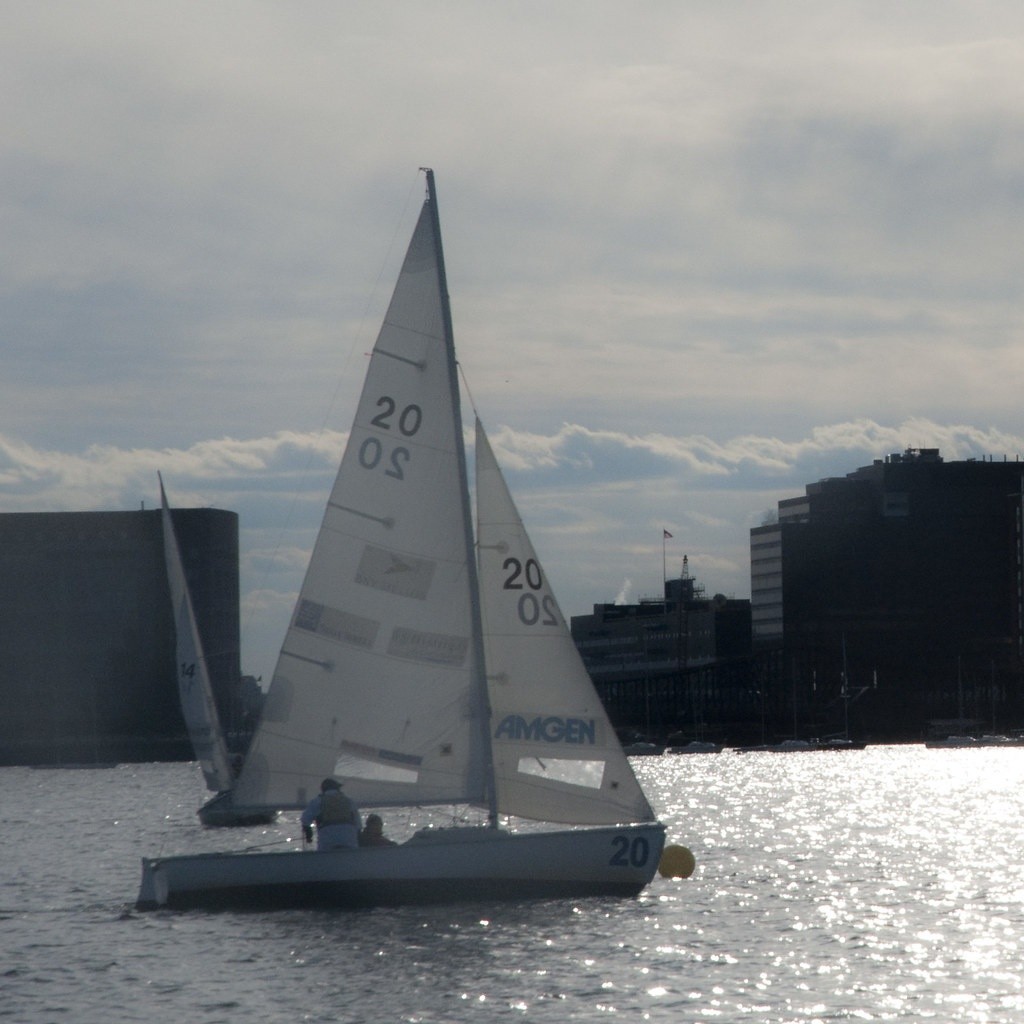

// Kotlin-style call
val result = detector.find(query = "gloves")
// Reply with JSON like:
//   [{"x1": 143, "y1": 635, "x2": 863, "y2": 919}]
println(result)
[{"x1": 305, "y1": 828, "x2": 314, "y2": 843}]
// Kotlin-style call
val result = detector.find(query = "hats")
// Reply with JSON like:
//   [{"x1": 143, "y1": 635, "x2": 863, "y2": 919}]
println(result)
[
  {"x1": 365, "y1": 814, "x2": 382, "y2": 822},
  {"x1": 322, "y1": 779, "x2": 339, "y2": 789}
]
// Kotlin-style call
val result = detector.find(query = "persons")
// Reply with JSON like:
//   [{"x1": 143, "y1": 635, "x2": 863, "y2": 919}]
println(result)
[{"x1": 301, "y1": 777, "x2": 362, "y2": 850}]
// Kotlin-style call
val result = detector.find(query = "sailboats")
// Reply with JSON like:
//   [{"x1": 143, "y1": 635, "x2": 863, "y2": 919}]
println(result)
[
  {"x1": 623, "y1": 630, "x2": 1023, "y2": 754},
  {"x1": 138, "y1": 168, "x2": 670, "y2": 908},
  {"x1": 155, "y1": 467, "x2": 280, "y2": 828}
]
